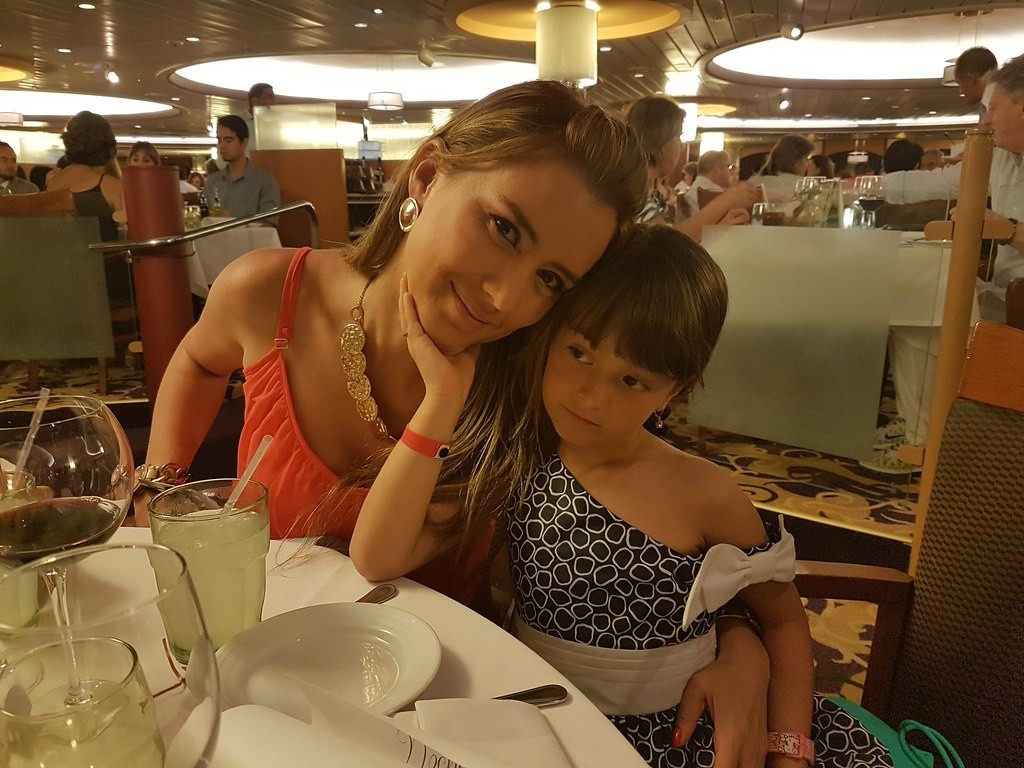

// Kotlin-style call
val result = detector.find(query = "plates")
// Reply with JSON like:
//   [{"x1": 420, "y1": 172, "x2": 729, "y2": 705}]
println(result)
[{"x1": 203, "y1": 602, "x2": 442, "y2": 716}]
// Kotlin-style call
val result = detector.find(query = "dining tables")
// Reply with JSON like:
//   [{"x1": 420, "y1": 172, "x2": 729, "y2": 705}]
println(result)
[{"x1": 0, "y1": 526, "x2": 652, "y2": 768}]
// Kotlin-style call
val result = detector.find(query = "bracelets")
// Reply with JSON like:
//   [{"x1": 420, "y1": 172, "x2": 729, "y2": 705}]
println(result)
[
  {"x1": 402, "y1": 425, "x2": 449, "y2": 459},
  {"x1": 999, "y1": 217, "x2": 1018, "y2": 246},
  {"x1": 768, "y1": 732, "x2": 814, "y2": 766},
  {"x1": 715, "y1": 609, "x2": 761, "y2": 634}
]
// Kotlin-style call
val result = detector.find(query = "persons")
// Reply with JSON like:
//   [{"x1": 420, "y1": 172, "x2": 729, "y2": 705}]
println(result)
[
  {"x1": 348, "y1": 223, "x2": 814, "y2": 767},
  {"x1": 0, "y1": 141, "x2": 52, "y2": 194},
  {"x1": 203, "y1": 115, "x2": 281, "y2": 228},
  {"x1": 180, "y1": 160, "x2": 220, "y2": 191},
  {"x1": 674, "y1": 151, "x2": 739, "y2": 217},
  {"x1": 133, "y1": 82, "x2": 773, "y2": 768},
  {"x1": 807, "y1": 154, "x2": 835, "y2": 178},
  {"x1": 627, "y1": 95, "x2": 757, "y2": 242},
  {"x1": 883, "y1": 139, "x2": 946, "y2": 173},
  {"x1": 46, "y1": 111, "x2": 127, "y2": 298},
  {"x1": 129, "y1": 141, "x2": 159, "y2": 166},
  {"x1": 859, "y1": 53, "x2": 1024, "y2": 474},
  {"x1": 954, "y1": 46, "x2": 998, "y2": 160},
  {"x1": 747, "y1": 134, "x2": 814, "y2": 204},
  {"x1": 217, "y1": 84, "x2": 275, "y2": 169}
]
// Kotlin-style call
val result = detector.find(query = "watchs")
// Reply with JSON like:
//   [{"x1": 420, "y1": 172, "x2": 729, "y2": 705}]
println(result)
[{"x1": 133, "y1": 463, "x2": 190, "y2": 496}]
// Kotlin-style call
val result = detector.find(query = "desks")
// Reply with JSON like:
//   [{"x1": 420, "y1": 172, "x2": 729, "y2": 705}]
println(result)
[{"x1": 184, "y1": 227, "x2": 279, "y2": 325}]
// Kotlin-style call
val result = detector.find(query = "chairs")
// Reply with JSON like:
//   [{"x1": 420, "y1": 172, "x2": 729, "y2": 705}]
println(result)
[
  {"x1": 794, "y1": 319, "x2": 1024, "y2": 768},
  {"x1": 0, "y1": 189, "x2": 139, "y2": 397},
  {"x1": 697, "y1": 185, "x2": 767, "y2": 211}
]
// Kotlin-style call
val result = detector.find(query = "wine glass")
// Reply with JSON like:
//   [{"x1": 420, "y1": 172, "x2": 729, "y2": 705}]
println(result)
[
  {"x1": 796, "y1": 175, "x2": 888, "y2": 228},
  {"x1": 0, "y1": 394, "x2": 159, "y2": 713}
]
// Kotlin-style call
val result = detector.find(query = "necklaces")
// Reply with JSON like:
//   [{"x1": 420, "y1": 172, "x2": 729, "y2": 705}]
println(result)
[{"x1": 341, "y1": 268, "x2": 398, "y2": 445}]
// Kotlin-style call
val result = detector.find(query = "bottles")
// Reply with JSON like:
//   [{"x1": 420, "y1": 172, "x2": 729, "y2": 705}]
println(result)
[
  {"x1": 200, "y1": 188, "x2": 210, "y2": 218},
  {"x1": 212, "y1": 188, "x2": 223, "y2": 216}
]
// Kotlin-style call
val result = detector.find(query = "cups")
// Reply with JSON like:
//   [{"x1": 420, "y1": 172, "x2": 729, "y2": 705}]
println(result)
[
  {"x1": 752, "y1": 202, "x2": 785, "y2": 227},
  {"x1": 0, "y1": 542, "x2": 222, "y2": 768},
  {"x1": 146, "y1": 478, "x2": 271, "y2": 664}
]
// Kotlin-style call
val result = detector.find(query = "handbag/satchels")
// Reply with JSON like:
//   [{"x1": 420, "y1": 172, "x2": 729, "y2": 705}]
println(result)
[{"x1": 826, "y1": 693, "x2": 963, "y2": 768}]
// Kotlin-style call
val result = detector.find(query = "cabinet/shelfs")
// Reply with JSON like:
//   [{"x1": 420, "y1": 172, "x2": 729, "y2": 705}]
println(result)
[{"x1": 248, "y1": 149, "x2": 411, "y2": 247}]
